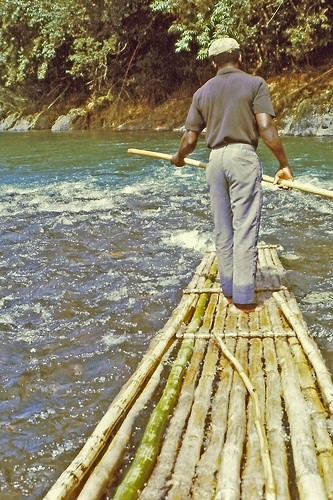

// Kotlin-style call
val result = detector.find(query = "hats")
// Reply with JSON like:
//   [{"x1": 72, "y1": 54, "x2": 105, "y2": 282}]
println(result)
[{"x1": 209, "y1": 38, "x2": 240, "y2": 58}]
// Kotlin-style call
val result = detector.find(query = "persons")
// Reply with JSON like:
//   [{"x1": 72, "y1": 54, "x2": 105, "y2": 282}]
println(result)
[{"x1": 170, "y1": 38, "x2": 294, "y2": 310}]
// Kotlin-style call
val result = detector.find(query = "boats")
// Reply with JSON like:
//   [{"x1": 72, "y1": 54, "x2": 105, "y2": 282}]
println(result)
[{"x1": 42, "y1": 239, "x2": 333, "y2": 500}]
